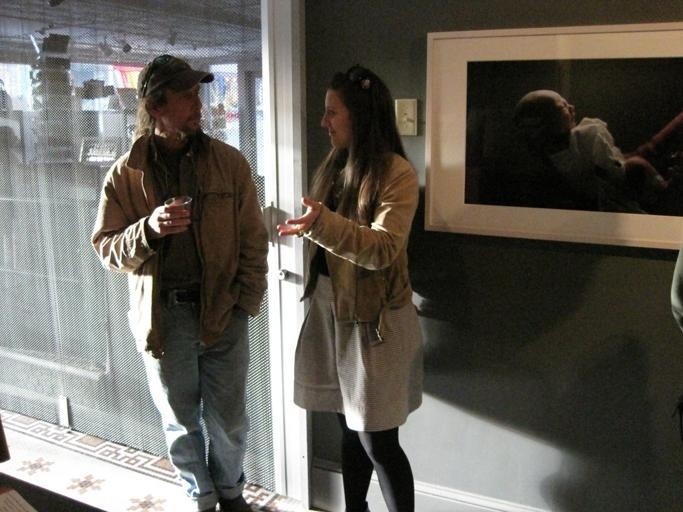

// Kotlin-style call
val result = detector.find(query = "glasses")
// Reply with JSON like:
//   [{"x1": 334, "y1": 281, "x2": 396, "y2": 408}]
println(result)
[
  {"x1": 347, "y1": 65, "x2": 372, "y2": 91},
  {"x1": 141, "y1": 53, "x2": 172, "y2": 98}
]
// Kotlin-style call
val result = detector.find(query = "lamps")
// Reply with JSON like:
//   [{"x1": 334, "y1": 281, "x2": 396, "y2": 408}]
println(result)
[{"x1": 95, "y1": 31, "x2": 132, "y2": 59}]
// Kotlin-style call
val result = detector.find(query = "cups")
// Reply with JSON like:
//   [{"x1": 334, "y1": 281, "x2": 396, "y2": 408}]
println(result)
[{"x1": 164, "y1": 196, "x2": 193, "y2": 225}]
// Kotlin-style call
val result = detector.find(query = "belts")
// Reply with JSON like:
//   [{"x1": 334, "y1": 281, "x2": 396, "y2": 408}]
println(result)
[{"x1": 159, "y1": 286, "x2": 201, "y2": 309}]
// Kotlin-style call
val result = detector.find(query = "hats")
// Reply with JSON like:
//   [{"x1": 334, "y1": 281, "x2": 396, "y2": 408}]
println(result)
[{"x1": 137, "y1": 53, "x2": 215, "y2": 98}]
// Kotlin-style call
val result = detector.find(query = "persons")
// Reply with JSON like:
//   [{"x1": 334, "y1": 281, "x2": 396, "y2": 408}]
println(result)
[
  {"x1": 511, "y1": 91, "x2": 682, "y2": 214},
  {"x1": 89, "y1": 55, "x2": 269, "y2": 512},
  {"x1": 275, "y1": 64, "x2": 423, "y2": 512}
]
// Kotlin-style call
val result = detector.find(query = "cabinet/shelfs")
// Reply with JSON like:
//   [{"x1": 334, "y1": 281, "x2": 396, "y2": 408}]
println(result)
[{"x1": 0, "y1": 103, "x2": 137, "y2": 428}]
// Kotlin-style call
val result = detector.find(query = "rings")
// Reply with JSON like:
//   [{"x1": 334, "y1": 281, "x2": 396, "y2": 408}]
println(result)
[{"x1": 296, "y1": 226, "x2": 305, "y2": 238}]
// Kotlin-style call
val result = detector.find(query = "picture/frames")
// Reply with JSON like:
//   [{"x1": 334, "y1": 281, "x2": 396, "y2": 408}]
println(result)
[{"x1": 423, "y1": 20, "x2": 683, "y2": 255}]
